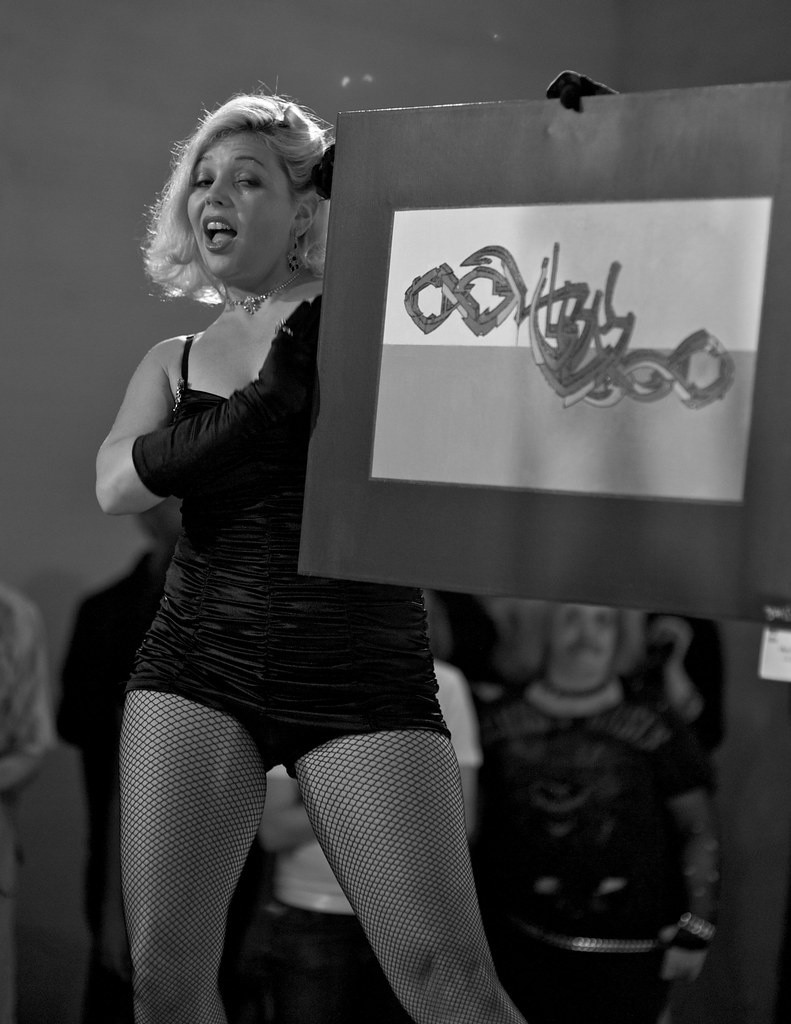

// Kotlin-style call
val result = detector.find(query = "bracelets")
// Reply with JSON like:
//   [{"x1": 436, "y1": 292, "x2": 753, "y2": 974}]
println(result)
[{"x1": 678, "y1": 912, "x2": 717, "y2": 943}]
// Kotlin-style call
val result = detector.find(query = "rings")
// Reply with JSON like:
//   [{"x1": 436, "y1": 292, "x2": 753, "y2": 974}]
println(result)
[{"x1": 274, "y1": 318, "x2": 294, "y2": 337}]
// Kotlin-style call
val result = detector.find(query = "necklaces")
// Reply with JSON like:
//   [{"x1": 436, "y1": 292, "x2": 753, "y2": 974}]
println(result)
[{"x1": 226, "y1": 271, "x2": 299, "y2": 316}]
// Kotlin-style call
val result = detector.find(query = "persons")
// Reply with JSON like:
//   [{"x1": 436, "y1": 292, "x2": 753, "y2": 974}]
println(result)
[
  {"x1": 96, "y1": 68, "x2": 621, "y2": 1024},
  {"x1": 0, "y1": 492, "x2": 727, "y2": 1024}
]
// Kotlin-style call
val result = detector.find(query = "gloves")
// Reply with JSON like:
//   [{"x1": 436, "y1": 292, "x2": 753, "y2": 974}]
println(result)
[
  {"x1": 132, "y1": 293, "x2": 324, "y2": 497},
  {"x1": 546, "y1": 69, "x2": 621, "y2": 114}
]
